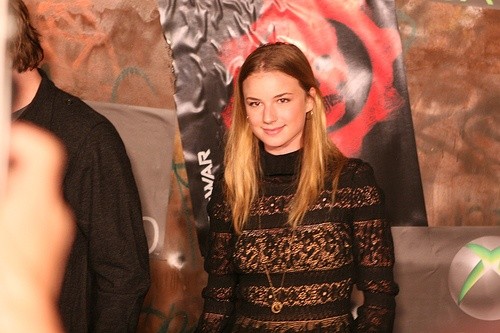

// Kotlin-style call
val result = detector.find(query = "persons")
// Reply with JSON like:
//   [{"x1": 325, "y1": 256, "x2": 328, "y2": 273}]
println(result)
[
  {"x1": 0, "y1": 1, "x2": 150, "y2": 332},
  {"x1": 198, "y1": 42, "x2": 398, "y2": 333}
]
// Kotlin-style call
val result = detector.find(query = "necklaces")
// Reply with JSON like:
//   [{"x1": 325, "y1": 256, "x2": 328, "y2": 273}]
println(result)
[{"x1": 259, "y1": 186, "x2": 296, "y2": 314}]
